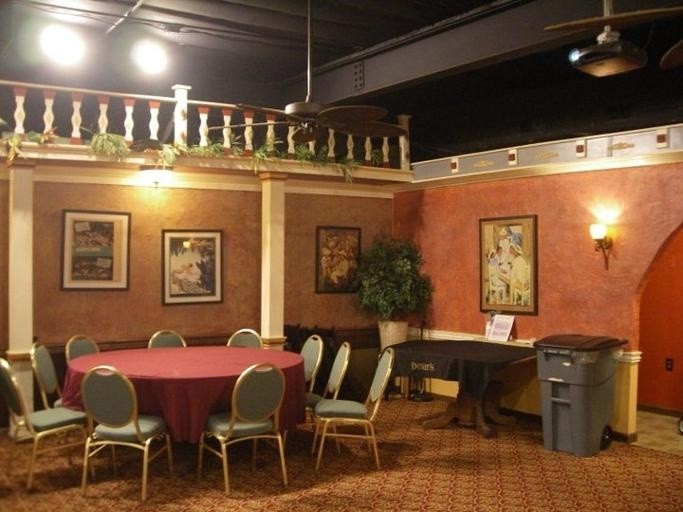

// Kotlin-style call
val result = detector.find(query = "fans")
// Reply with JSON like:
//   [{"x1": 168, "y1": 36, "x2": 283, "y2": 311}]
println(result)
[
  {"x1": 209, "y1": 4, "x2": 411, "y2": 143},
  {"x1": 544, "y1": 4, "x2": 683, "y2": 71}
]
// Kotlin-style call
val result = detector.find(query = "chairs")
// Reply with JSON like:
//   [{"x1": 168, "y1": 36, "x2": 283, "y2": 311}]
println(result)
[
  {"x1": 28, "y1": 342, "x2": 64, "y2": 408},
  {"x1": 297, "y1": 341, "x2": 350, "y2": 453},
  {"x1": 0, "y1": 356, "x2": 95, "y2": 491},
  {"x1": 81, "y1": 365, "x2": 174, "y2": 500},
  {"x1": 197, "y1": 363, "x2": 291, "y2": 495},
  {"x1": 300, "y1": 333, "x2": 324, "y2": 392},
  {"x1": 227, "y1": 329, "x2": 262, "y2": 347},
  {"x1": 310, "y1": 346, "x2": 394, "y2": 472},
  {"x1": 148, "y1": 329, "x2": 186, "y2": 347},
  {"x1": 65, "y1": 334, "x2": 99, "y2": 359}
]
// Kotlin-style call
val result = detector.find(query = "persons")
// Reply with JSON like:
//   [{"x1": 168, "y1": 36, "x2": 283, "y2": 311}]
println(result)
[
  {"x1": 321, "y1": 231, "x2": 359, "y2": 284},
  {"x1": 487, "y1": 225, "x2": 530, "y2": 307}
]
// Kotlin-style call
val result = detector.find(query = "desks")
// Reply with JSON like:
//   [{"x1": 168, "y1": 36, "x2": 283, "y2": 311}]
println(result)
[
  {"x1": 62, "y1": 346, "x2": 305, "y2": 449},
  {"x1": 385, "y1": 340, "x2": 538, "y2": 436}
]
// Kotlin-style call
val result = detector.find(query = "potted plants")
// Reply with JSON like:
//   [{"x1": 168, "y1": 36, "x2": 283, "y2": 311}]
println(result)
[{"x1": 356, "y1": 232, "x2": 434, "y2": 353}]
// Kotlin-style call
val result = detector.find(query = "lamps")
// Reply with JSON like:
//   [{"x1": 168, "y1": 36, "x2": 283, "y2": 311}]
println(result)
[{"x1": 590, "y1": 224, "x2": 613, "y2": 269}]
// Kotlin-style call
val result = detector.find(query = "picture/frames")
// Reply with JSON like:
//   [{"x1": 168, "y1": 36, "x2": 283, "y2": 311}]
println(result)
[
  {"x1": 61, "y1": 209, "x2": 130, "y2": 291},
  {"x1": 480, "y1": 216, "x2": 539, "y2": 315},
  {"x1": 315, "y1": 225, "x2": 362, "y2": 294},
  {"x1": 163, "y1": 230, "x2": 224, "y2": 304}
]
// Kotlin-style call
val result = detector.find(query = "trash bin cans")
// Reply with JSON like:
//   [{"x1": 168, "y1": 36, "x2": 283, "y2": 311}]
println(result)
[{"x1": 533, "y1": 334, "x2": 629, "y2": 456}]
